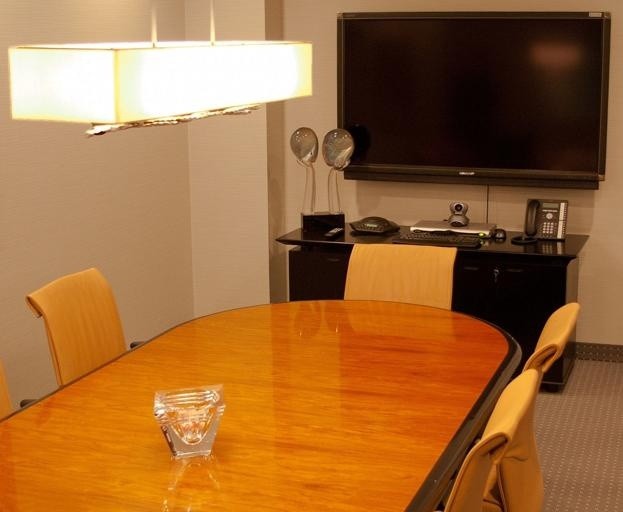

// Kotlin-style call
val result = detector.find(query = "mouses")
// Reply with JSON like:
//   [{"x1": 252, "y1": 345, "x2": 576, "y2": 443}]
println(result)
[{"x1": 493, "y1": 227, "x2": 506, "y2": 240}]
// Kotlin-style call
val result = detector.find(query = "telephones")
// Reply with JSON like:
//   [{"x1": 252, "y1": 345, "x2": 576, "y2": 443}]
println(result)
[
  {"x1": 349, "y1": 217, "x2": 400, "y2": 233},
  {"x1": 523, "y1": 199, "x2": 568, "y2": 241}
]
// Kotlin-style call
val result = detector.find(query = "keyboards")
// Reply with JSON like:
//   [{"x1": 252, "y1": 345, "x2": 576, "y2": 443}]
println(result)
[{"x1": 393, "y1": 233, "x2": 479, "y2": 249}]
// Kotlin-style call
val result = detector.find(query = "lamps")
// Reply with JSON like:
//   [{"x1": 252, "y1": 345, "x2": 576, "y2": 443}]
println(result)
[{"x1": 8, "y1": 0, "x2": 313, "y2": 138}]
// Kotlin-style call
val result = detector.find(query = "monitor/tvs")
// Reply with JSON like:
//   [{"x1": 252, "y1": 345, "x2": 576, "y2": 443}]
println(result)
[{"x1": 335, "y1": 12, "x2": 610, "y2": 187}]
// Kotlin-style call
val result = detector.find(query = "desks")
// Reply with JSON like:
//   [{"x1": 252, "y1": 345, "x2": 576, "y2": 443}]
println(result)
[
  {"x1": 275, "y1": 224, "x2": 589, "y2": 392},
  {"x1": 0, "y1": 299, "x2": 521, "y2": 512}
]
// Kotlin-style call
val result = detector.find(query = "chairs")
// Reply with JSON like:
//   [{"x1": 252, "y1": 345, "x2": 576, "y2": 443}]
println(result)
[
  {"x1": 437, "y1": 301, "x2": 582, "y2": 512},
  {"x1": 27, "y1": 267, "x2": 144, "y2": 386},
  {"x1": 0, "y1": 359, "x2": 38, "y2": 421},
  {"x1": 344, "y1": 244, "x2": 457, "y2": 310}
]
